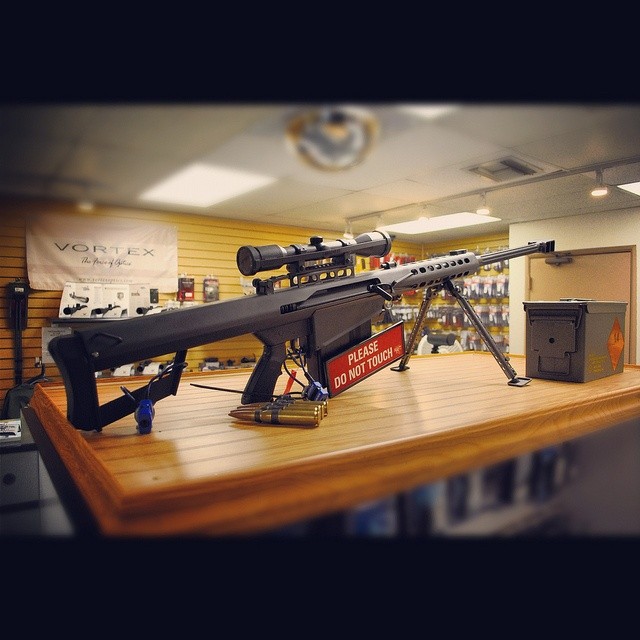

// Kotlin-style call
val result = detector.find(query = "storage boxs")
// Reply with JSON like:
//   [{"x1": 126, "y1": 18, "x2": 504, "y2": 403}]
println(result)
[{"x1": 522, "y1": 297, "x2": 629, "y2": 384}]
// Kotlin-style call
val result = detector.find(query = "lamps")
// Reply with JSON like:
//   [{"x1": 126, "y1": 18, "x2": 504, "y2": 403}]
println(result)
[
  {"x1": 476, "y1": 191, "x2": 491, "y2": 215},
  {"x1": 376, "y1": 213, "x2": 384, "y2": 230},
  {"x1": 418, "y1": 204, "x2": 429, "y2": 223},
  {"x1": 285, "y1": 104, "x2": 379, "y2": 173},
  {"x1": 591, "y1": 168, "x2": 607, "y2": 197},
  {"x1": 343, "y1": 221, "x2": 353, "y2": 239}
]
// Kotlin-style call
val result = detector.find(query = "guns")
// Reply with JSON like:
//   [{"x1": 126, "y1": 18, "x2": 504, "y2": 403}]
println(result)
[{"x1": 48, "y1": 230, "x2": 554, "y2": 432}]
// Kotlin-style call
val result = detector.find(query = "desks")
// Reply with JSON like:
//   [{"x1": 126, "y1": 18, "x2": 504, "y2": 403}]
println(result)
[{"x1": 28, "y1": 348, "x2": 640, "y2": 539}]
